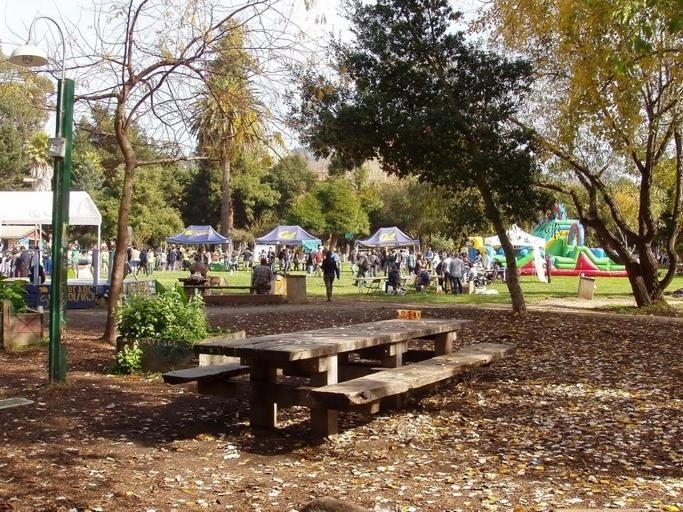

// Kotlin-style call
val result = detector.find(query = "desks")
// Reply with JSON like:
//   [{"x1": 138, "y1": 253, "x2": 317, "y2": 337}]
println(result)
[
  {"x1": 191, "y1": 314, "x2": 477, "y2": 442},
  {"x1": 353, "y1": 268, "x2": 508, "y2": 295}
]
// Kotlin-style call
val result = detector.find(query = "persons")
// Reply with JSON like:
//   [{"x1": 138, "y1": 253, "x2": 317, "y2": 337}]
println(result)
[
  {"x1": 544, "y1": 256, "x2": 552, "y2": 283},
  {"x1": 0, "y1": 243, "x2": 502, "y2": 301}
]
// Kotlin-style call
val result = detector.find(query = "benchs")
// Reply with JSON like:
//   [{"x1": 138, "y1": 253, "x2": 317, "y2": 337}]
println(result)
[
  {"x1": 308, "y1": 341, "x2": 518, "y2": 416},
  {"x1": 161, "y1": 359, "x2": 250, "y2": 398}
]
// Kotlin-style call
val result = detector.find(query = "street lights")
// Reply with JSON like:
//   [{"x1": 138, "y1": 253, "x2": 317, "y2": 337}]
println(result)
[{"x1": 8, "y1": 17, "x2": 75, "y2": 382}]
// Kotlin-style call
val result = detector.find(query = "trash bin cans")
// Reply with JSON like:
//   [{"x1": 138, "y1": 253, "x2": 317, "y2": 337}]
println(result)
[
  {"x1": 578, "y1": 277, "x2": 595, "y2": 300},
  {"x1": 286, "y1": 275, "x2": 307, "y2": 304}
]
